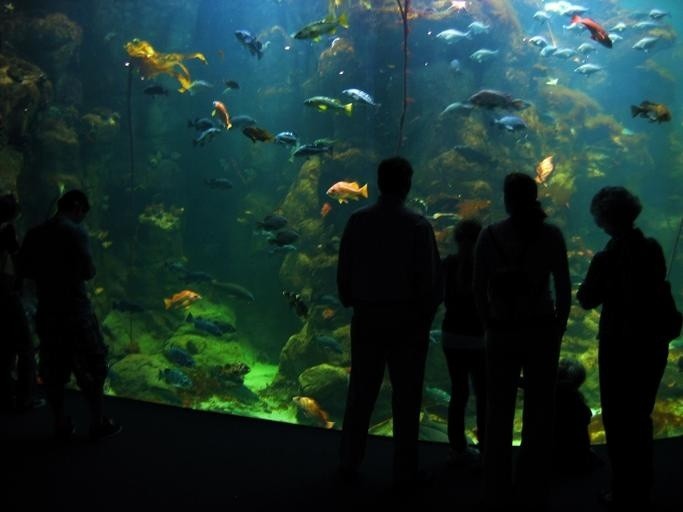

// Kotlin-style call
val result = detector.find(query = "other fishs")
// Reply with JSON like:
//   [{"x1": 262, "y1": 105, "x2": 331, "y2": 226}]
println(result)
[{"x1": 2, "y1": 2, "x2": 683, "y2": 444}]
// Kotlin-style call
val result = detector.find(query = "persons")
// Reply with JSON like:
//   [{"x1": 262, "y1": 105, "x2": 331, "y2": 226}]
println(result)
[
  {"x1": 436, "y1": 220, "x2": 492, "y2": 462},
  {"x1": 20, "y1": 190, "x2": 109, "y2": 460},
  {"x1": 473, "y1": 171, "x2": 575, "y2": 496},
  {"x1": 518, "y1": 359, "x2": 593, "y2": 465},
  {"x1": 578, "y1": 186, "x2": 683, "y2": 478},
  {"x1": 338, "y1": 154, "x2": 445, "y2": 478},
  {"x1": 0, "y1": 191, "x2": 45, "y2": 422}
]
